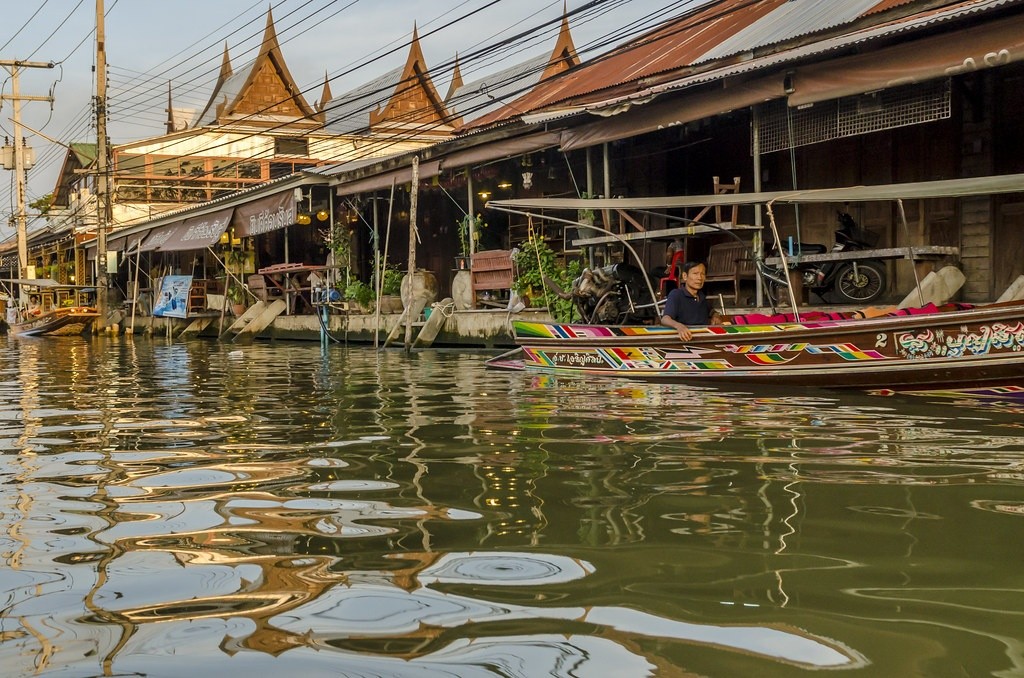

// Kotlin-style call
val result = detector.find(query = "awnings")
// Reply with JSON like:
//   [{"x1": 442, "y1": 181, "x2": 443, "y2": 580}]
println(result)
[
  {"x1": 86, "y1": 205, "x2": 234, "y2": 262},
  {"x1": 336, "y1": 159, "x2": 443, "y2": 197},
  {"x1": 442, "y1": 127, "x2": 564, "y2": 171},
  {"x1": 559, "y1": 68, "x2": 794, "y2": 153},
  {"x1": 787, "y1": 10, "x2": 1024, "y2": 110},
  {"x1": 233, "y1": 187, "x2": 298, "y2": 241}
]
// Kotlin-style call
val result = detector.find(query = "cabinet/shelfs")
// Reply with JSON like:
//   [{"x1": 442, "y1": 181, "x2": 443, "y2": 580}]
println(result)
[{"x1": 187, "y1": 278, "x2": 208, "y2": 314}]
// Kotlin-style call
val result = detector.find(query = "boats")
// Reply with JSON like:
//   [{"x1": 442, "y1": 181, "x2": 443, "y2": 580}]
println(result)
[
  {"x1": 0, "y1": 277, "x2": 102, "y2": 338},
  {"x1": 508, "y1": 300, "x2": 984, "y2": 342},
  {"x1": 522, "y1": 302, "x2": 1024, "y2": 390}
]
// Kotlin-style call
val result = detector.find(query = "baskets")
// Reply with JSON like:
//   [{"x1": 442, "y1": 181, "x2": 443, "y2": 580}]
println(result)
[{"x1": 857, "y1": 230, "x2": 880, "y2": 247}]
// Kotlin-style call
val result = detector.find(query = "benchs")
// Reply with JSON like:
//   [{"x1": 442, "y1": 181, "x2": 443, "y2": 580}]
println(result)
[
  {"x1": 676, "y1": 239, "x2": 764, "y2": 308},
  {"x1": 471, "y1": 248, "x2": 544, "y2": 308}
]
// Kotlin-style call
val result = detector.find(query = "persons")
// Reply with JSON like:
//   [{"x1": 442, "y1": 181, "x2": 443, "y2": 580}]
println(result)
[
  {"x1": 24, "y1": 295, "x2": 41, "y2": 315},
  {"x1": 666, "y1": 234, "x2": 683, "y2": 280},
  {"x1": 80, "y1": 279, "x2": 97, "y2": 304},
  {"x1": 660, "y1": 257, "x2": 724, "y2": 341}
]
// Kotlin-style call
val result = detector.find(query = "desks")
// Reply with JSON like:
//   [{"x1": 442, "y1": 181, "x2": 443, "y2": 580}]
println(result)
[{"x1": 259, "y1": 266, "x2": 336, "y2": 315}]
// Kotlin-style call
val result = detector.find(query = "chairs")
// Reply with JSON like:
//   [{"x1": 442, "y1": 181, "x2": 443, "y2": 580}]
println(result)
[{"x1": 658, "y1": 249, "x2": 684, "y2": 301}]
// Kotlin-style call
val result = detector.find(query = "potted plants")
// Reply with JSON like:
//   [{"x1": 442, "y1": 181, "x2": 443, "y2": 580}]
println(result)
[
  {"x1": 374, "y1": 268, "x2": 405, "y2": 316},
  {"x1": 226, "y1": 286, "x2": 245, "y2": 316}
]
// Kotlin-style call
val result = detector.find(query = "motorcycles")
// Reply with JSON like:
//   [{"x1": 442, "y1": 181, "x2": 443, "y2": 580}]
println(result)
[{"x1": 759, "y1": 200, "x2": 889, "y2": 304}]
